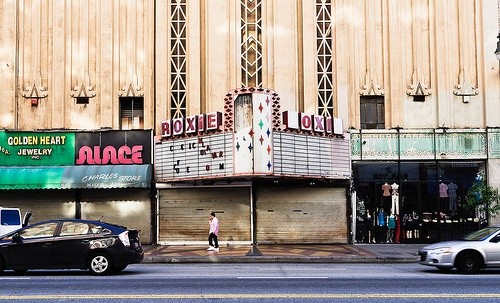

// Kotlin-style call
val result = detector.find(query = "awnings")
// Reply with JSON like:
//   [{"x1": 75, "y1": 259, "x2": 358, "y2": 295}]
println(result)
[{"x1": 0, "y1": 164, "x2": 153, "y2": 190}]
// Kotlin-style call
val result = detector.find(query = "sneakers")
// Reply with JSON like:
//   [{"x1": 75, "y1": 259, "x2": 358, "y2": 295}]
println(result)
[
  {"x1": 214, "y1": 247, "x2": 219, "y2": 251},
  {"x1": 207, "y1": 246, "x2": 214, "y2": 250}
]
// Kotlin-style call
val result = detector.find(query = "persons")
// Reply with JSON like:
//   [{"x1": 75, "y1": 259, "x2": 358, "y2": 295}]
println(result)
[
  {"x1": 366, "y1": 208, "x2": 401, "y2": 244},
  {"x1": 381, "y1": 182, "x2": 391, "y2": 213},
  {"x1": 390, "y1": 182, "x2": 400, "y2": 215},
  {"x1": 439, "y1": 182, "x2": 448, "y2": 212},
  {"x1": 405, "y1": 210, "x2": 420, "y2": 238},
  {"x1": 206, "y1": 212, "x2": 219, "y2": 252},
  {"x1": 448, "y1": 182, "x2": 458, "y2": 212}
]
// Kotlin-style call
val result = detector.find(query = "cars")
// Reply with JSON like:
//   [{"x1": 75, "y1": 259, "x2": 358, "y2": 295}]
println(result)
[
  {"x1": 1, "y1": 219, "x2": 144, "y2": 275},
  {"x1": 417, "y1": 225, "x2": 500, "y2": 275}
]
